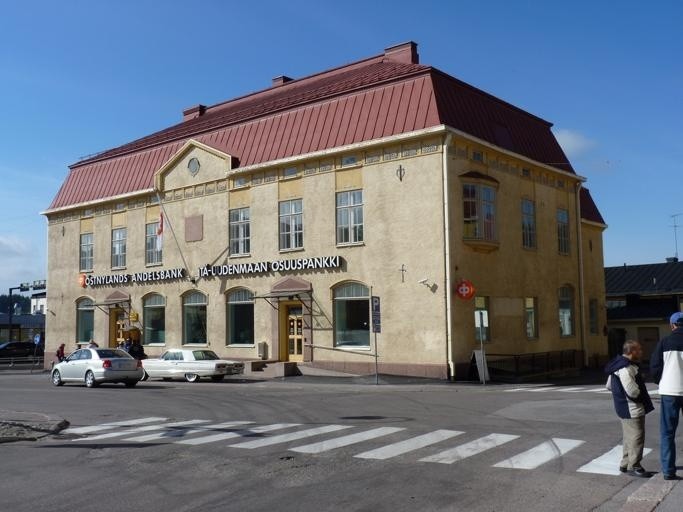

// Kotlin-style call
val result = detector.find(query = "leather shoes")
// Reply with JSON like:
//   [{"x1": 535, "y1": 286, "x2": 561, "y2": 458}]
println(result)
[{"x1": 620, "y1": 466, "x2": 681, "y2": 480}]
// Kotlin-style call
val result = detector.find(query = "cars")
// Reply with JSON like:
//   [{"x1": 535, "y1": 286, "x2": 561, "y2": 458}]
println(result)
[
  {"x1": 50, "y1": 347, "x2": 144, "y2": 389},
  {"x1": 0, "y1": 340, "x2": 45, "y2": 363},
  {"x1": 140, "y1": 346, "x2": 246, "y2": 383}
]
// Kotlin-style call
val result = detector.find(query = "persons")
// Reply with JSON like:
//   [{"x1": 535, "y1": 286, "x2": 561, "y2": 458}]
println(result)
[
  {"x1": 56, "y1": 343, "x2": 64, "y2": 362},
  {"x1": 74, "y1": 338, "x2": 146, "y2": 359},
  {"x1": 649, "y1": 310, "x2": 683, "y2": 481},
  {"x1": 603, "y1": 339, "x2": 655, "y2": 478}
]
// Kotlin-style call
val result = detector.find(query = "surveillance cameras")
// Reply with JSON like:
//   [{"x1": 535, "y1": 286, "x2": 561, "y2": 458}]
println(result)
[
  {"x1": 418, "y1": 278, "x2": 427, "y2": 283},
  {"x1": 45, "y1": 307, "x2": 49, "y2": 312}
]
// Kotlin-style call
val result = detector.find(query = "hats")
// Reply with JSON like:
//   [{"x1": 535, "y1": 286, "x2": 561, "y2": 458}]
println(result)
[{"x1": 669, "y1": 311, "x2": 683, "y2": 324}]
